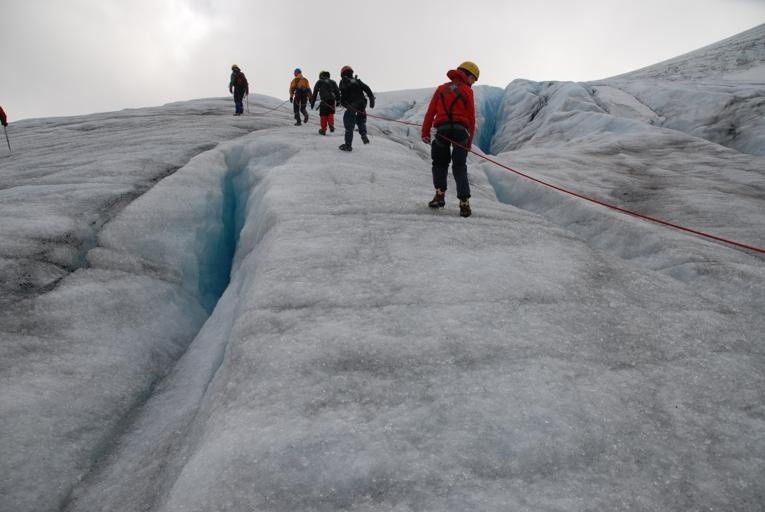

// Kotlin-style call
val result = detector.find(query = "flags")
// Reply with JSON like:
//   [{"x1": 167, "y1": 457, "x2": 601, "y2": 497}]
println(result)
[{"x1": 0, "y1": 107, "x2": 8, "y2": 127}]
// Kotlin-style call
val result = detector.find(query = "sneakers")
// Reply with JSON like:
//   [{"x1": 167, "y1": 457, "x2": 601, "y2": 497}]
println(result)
[
  {"x1": 319, "y1": 125, "x2": 334, "y2": 135},
  {"x1": 295, "y1": 115, "x2": 308, "y2": 125},
  {"x1": 361, "y1": 134, "x2": 369, "y2": 144},
  {"x1": 340, "y1": 144, "x2": 352, "y2": 151},
  {"x1": 459, "y1": 205, "x2": 471, "y2": 217},
  {"x1": 428, "y1": 194, "x2": 445, "y2": 207},
  {"x1": 233, "y1": 111, "x2": 243, "y2": 116}
]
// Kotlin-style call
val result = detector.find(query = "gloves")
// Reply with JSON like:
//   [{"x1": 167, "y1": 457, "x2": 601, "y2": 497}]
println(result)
[
  {"x1": 311, "y1": 100, "x2": 315, "y2": 108},
  {"x1": 336, "y1": 102, "x2": 340, "y2": 106},
  {"x1": 370, "y1": 99, "x2": 375, "y2": 107},
  {"x1": 290, "y1": 97, "x2": 293, "y2": 103}
]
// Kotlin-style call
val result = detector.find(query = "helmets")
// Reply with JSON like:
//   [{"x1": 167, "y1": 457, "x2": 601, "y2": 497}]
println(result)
[
  {"x1": 457, "y1": 62, "x2": 479, "y2": 81},
  {"x1": 341, "y1": 66, "x2": 353, "y2": 76},
  {"x1": 232, "y1": 64, "x2": 237, "y2": 69},
  {"x1": 295, "y1": 67, "x2": 301, "y2": 72}
]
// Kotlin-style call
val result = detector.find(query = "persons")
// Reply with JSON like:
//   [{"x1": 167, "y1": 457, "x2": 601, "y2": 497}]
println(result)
[
  {"x1": 421, "y1": 61, "x2": 481, "y2": 218},
  {"x1": 229, "y1": 64, "x2": 250, "y2": 116},
  {"x1": 337, "y1": 65, "x2": 376, "y2": 152},
  {"x1": 289, "y1": 66, "x2": 313, "y2": 126},
  {"x1": 310, "y1": 70, "x2": 342, "y2": 136}
]
